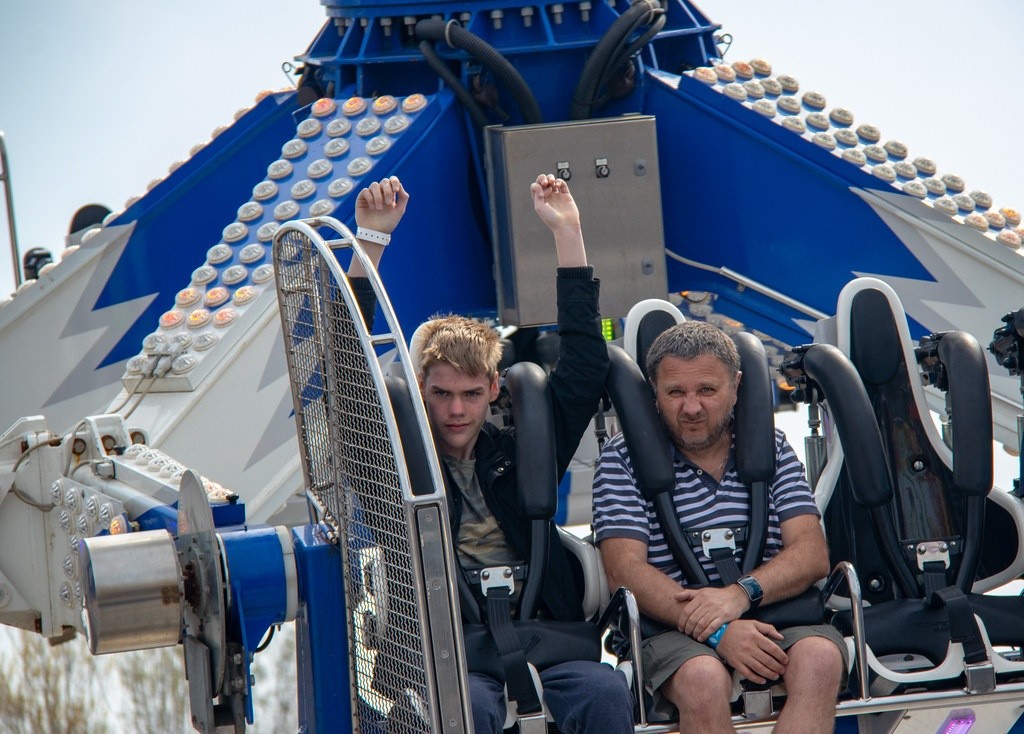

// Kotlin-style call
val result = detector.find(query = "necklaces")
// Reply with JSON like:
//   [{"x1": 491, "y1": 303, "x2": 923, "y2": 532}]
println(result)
[{"x1": 711, "y1": 431, "x2": 729, "y2": 477}]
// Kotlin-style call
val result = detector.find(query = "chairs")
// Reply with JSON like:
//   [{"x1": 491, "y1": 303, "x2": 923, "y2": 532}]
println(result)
[
  {"x1": 597, "y1": 291, "x2": 857, "y2": 722},
  {"x1": 378, "y1": 326, "x2": 642, "y2": 734},
  {"x1": 806, "y1": 269, "x2": 1024, "y2": 696}
]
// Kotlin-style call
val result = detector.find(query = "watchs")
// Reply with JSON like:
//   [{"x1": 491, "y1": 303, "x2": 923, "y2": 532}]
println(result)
[{"x1": 736, "y1": 574, "x2": 764, "y2": 613}]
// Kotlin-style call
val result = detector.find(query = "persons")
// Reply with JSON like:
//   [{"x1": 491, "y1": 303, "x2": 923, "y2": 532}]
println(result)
[
  {"x1": 592, "y1": 321, "x2": 849, "y2": 734},
  {"x1": 321, "y1": 174, "x2": 634, "y2": 734}
]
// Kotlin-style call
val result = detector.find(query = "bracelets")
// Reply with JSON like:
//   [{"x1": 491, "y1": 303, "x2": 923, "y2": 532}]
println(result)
[
  {"x1": 706, "y1": 624, "x2": 728, "y2": 650},
  {"x1": 355, "y1": 226, "x2": 391, "y2": 246}
]
